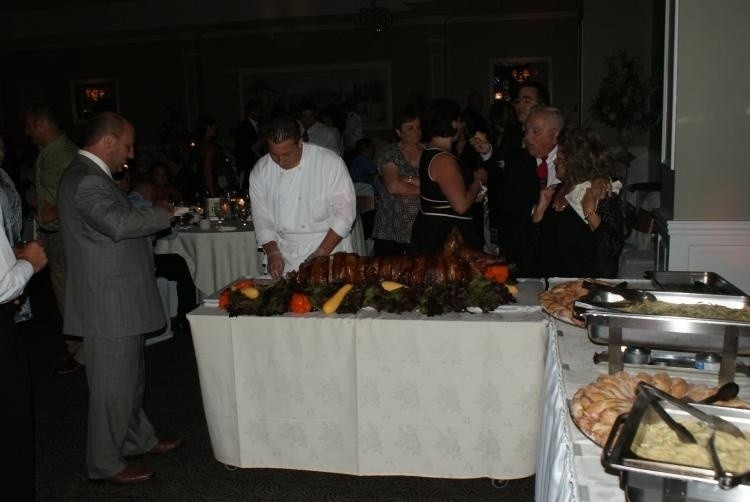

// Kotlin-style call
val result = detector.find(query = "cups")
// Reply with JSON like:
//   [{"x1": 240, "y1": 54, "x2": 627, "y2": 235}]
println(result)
[{"x1": 199, "y1": 219, "x2": 210, "y2": 230}]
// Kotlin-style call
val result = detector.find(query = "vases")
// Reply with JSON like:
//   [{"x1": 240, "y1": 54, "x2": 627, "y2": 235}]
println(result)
[{"x1": 616, "y1": 139, "x2": 636, "y2": 167}]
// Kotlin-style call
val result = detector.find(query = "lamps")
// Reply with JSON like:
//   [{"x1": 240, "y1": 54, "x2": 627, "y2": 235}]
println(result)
[{"x1": 356, "y1": 1, "x2": 391, "y2": 33}]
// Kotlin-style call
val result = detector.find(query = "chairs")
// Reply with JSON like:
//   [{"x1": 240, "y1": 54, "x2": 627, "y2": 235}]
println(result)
[{"x1": 354, "y1": 182, "x2": 375, "y2": 213}]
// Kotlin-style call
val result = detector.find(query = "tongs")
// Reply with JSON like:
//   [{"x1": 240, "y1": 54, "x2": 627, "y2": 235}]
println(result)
[
  {"x1": 705, "y1": 429, "x2": 750, "y2": 490},
  {"x1": 681, "y1": 382, "x2": 740, "y2": 405},
  {"x1": 574, "y1": 297, "x2": 632, "y2": 314},
  {"x1": 581, "y1": 279, "x2": 657, "y2": 302},
  {"x1": 634, "y1": 381, "x2": 745, "y2": 445}
]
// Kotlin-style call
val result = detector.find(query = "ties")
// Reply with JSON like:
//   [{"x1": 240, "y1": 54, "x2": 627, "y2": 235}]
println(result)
[{"x1": 538, "y1": 155, "x2": 548, "y2": 188}]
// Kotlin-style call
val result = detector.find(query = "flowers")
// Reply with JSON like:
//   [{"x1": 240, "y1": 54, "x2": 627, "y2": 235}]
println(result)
[{"x1": 586, "y1": 49, "x2": 660, "y2": 138}]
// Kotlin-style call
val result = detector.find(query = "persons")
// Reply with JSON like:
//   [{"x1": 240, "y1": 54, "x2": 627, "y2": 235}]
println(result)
[
  {"x1": 2, "y1": 101, "x2": 225, "y2": 483},
  {"x1": 224, "y1": 84, "x2": 626, "y2": 278}
]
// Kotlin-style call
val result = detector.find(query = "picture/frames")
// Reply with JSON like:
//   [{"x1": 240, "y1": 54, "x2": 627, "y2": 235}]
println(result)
[
  {"x1": 69, "y1": 75, "x2": 122, "y2": 126},
  {"x1": 235, "y1": 60, "x2": 394, "y2": 124},
  {"x1": 489, "y1": 56, "x2": 554, "y2": 109}
]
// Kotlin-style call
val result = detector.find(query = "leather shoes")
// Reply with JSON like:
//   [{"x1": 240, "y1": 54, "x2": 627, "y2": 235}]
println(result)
[
  {"x1": 95, "y1": 464, "x2": 154, "y2": 484},
  {"x1": 124, "y1": 436, "x2": 188, "y2": 459}
]
